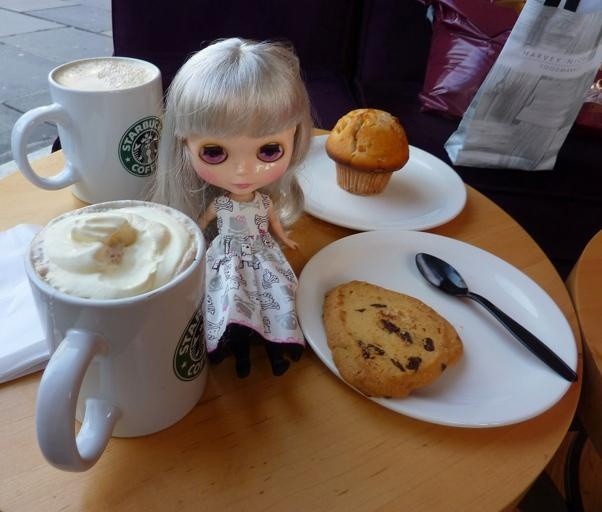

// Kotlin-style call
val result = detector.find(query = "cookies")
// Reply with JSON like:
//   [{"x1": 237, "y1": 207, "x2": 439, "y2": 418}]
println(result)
[{"x1": 322, "y1": 280, "x2": 463, "y2": 399}]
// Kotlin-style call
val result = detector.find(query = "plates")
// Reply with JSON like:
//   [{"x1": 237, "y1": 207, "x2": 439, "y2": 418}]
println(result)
[
  {"x1": 294, "y1": 134, "x2": 468, "y2": 231},
  {"x1": 295, "y1": 229, "x2": 578, "y2": 427}
]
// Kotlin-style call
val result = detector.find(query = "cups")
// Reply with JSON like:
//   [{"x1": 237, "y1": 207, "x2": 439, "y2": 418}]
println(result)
[
  {"x1": 10, "y1": 57, "x2": 165, "y2": 205},
  {"x1": 23, "y1": 199, "x2": 209, "y2": 472}
]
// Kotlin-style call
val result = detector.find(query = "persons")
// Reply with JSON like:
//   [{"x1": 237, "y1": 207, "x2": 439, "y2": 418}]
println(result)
[
  {"x1": 137, "y1": 137, "x2": 157, "y2": 163},
  {"x1": 134, "y1": 36, "x2": 322, "y2": 379}
]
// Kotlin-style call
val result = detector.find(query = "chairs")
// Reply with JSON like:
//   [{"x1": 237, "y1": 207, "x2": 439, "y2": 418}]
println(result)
[{"x1": 110, "y1": 0, "x2": 602, "y2": 279}]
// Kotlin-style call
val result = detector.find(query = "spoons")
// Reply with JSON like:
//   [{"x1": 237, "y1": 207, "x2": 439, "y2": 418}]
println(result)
[{"x1": 415, "y1": 252, "x2": 578, "y2": 382}]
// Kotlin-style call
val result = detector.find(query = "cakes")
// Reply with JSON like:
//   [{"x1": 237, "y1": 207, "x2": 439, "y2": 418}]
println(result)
[{"x1": 324, "y1": 108, "x2": 410, "y2": 196}]
